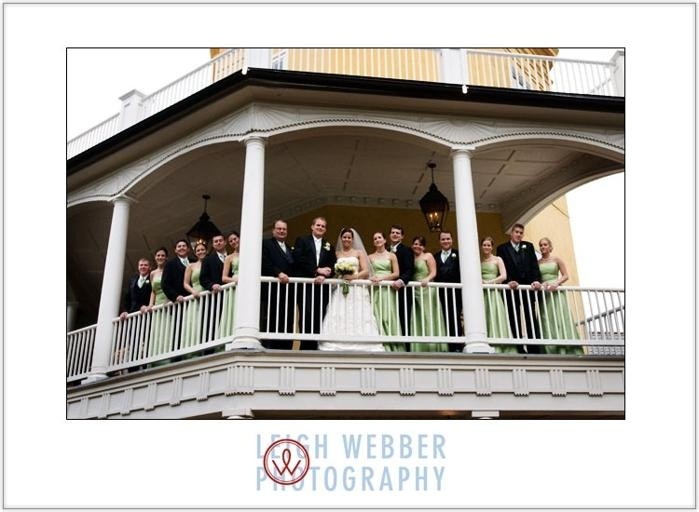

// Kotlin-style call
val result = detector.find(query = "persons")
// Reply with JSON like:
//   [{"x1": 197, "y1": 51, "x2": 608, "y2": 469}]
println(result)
[{"x1": 120, "y1": 217, "x2": 585, "y2": 373}]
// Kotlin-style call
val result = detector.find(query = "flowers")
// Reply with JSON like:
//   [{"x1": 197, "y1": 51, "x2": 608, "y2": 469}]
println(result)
[
  {"x1": 323, "y1": 241, "x2": 331, "y2": 251},
  {"x1": 521, "y1": 243, "x2": 529, "y2": 253},
  {"x1": 334, "y1": 259, "x2": 359, "y2": 294},
  {"x1": 143, "y1": 279, "x2": 151, "y2": 288},
  {"x1": 451, "y1": 251, "x2": 458, "y2": 263}
]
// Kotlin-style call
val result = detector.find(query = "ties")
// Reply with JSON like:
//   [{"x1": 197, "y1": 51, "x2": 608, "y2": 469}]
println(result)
[
  {"x1": 442, "y1": 251, "x2": 449, "y2": 263},
  {"x1": 515, "y1": 243, "x2": 520, "y2": 252},
  {"x1": 182, "y1": 258, "x2": 187, "y2": 266},
  {"x1": 222, "y1": 255, "x2": 226, "y2": 261}
]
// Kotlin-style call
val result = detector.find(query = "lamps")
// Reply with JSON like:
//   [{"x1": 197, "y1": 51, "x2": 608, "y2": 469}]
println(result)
[
  {"x1": 418, "y1": 162, "x2": 450, "y2": 232},
  {"x1": 185, "y1": 193, "x2": 221, "y2": 257}
]
